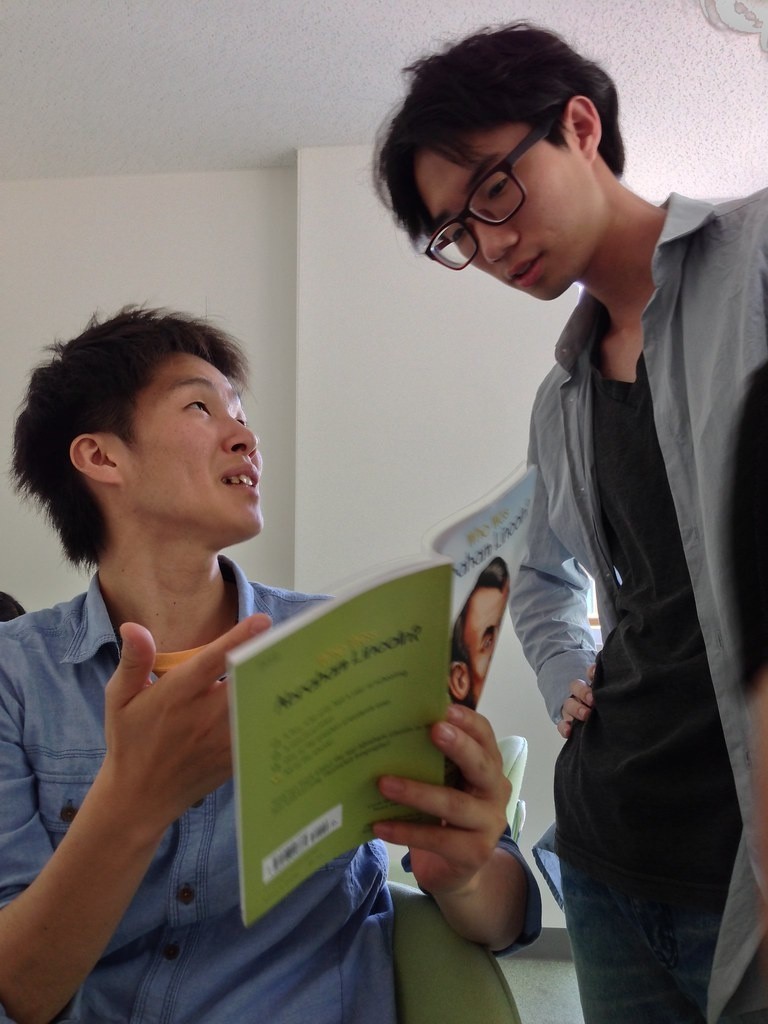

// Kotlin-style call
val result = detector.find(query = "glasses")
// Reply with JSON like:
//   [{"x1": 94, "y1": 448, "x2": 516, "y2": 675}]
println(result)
[{"x1": 424, "y1": 109, "x2": 558, "y2": 271}]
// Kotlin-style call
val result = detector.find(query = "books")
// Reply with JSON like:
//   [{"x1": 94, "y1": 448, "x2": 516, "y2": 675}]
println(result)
[{"x1": 229, "y1": 461, "x2": 538, "y2": 933}]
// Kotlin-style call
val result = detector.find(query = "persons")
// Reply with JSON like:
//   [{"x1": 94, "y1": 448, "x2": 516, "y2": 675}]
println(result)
[
  {"x1": 374, "y1": 15, "x2": 768, "y2": 1021},
  {"x1": 0, "y1": 309, "x2": 543, "y2": 1024}
]
